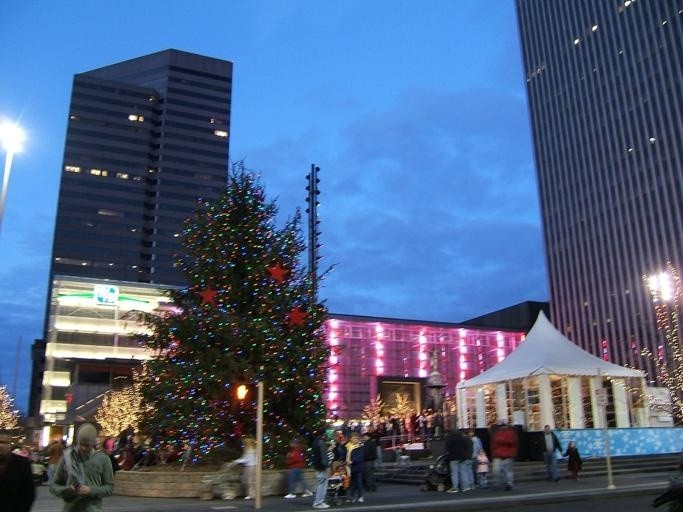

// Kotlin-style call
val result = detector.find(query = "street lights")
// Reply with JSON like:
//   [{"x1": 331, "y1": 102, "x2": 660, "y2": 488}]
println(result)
[{"x1": 238, "y1": 380, "x2": 264, "y2": 508}]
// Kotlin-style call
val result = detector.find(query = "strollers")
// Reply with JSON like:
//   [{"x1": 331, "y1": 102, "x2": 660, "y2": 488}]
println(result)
[
  {"x1": 321, "y1": 462, "x2": 347, "y2": 507},
  {"x1": 421, "y1": 453, "x2": 452, "y2": 492}
]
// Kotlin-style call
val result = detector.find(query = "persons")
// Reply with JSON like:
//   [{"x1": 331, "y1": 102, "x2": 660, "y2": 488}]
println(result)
[
  {"x1": 537, "y1": 424, "x2": 562, "y2": 481},
  {"x1": 50, "y1": 422, "x2": 116, "y2": 512},
  {"x1": 444, "y1": 427, "x2": 489, "y2": 492},
  {"x1": 493, "y1": 419, "x2": 519, "y2": 491},
  {"x1": 284, "y1": 430, "x2": 312, "y2": 498},
  {"x1": 311, "y1": 429, "x2": 331, "y2": 509},
  {"x1": 0, "y1": 429, "x2": 37, "y2": 511},
  {"x1": 332, "y1": 431, "x2": 377, "y2": 503},
  {"x1": 231, "y1": 436, "x2": 256, "y2": 500},
  {"x1": 562, "y1": 441, "x2": 583, "y2": 480}
]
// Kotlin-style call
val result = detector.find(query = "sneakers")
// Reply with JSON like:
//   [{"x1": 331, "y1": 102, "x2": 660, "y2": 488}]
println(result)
[
  {"x1": 302, "y1": 490, "x2": 314, "y2": 498},
  {"x1": 357, "y1": 497, "x2": 365, "y2": 502},
  {"x1": 345, "y1": 498, "x2": 355, "y2": 503},
  {"x1": 244, "y1": 495, "x2": 252, "y2": 500},
  {"x1": 313, "y1": 503, "x2": 330, "y2": 509},
  {"x1": 447, "y1": 489, "x2": 458, "y2": 493},
  {"x1": 462, "y1": 487, "x2": 471, "y2": 492},
  {"x1": 284, "y1": 493, "x2": 297, "y2": 499}
]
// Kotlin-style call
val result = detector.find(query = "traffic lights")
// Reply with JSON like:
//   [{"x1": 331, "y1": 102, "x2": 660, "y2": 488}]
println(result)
[{"x1": 234, "y1": 382, "x2": 255, "y2": 401}]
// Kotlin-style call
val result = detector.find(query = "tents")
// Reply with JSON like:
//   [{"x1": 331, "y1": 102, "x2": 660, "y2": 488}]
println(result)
[{"x1": 456, "y1": 310, "x2": 650, "y2": 430}]
